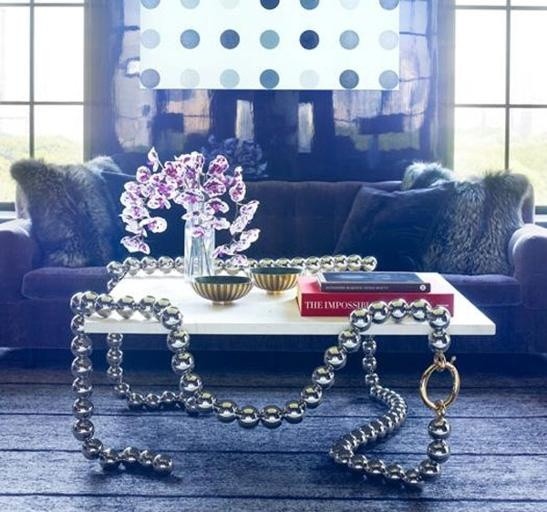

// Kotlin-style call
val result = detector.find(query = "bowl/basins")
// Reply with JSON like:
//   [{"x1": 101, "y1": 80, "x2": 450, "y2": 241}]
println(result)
[
  {"x1": 249, "y1": 267, "x2": 302, "y2": 296},
  {"x1": 190, "y1": 276, "x2": 255, "y2": 306}
]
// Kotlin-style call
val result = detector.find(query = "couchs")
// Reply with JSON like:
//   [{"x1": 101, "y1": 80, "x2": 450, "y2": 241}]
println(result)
[{"x1": 0, "y1": 170, "x2": 547, "y2": 354}]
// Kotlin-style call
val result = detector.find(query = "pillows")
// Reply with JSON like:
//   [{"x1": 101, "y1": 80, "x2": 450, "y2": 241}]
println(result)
[
  {"x1": 401, "y1": 159, "x2": 530, "y2": 277},
  {"x1": 10, "y1": 154, "x2": 125, "y2": 268},
  {"x1": 100, "y1": 170, "x2": 188, "y2": 260},
  {"x1": 333, "y1": 180, "x2": 457, "y2": 271}
]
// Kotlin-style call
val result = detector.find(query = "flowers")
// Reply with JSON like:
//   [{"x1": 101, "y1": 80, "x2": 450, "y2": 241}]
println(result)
[{"x1": 119, "y1": 146, "x2": 261, "y2": 271}]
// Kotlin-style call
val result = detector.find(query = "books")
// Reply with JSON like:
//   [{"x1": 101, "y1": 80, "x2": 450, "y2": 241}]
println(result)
[{"x1": 296, "y1": 269, "x2": 454, "y2": 320}]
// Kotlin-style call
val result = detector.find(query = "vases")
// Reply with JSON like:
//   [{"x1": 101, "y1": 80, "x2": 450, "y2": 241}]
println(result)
[{"x1": 184, "y1": 220, "x2": 216, "y2": 282}]
